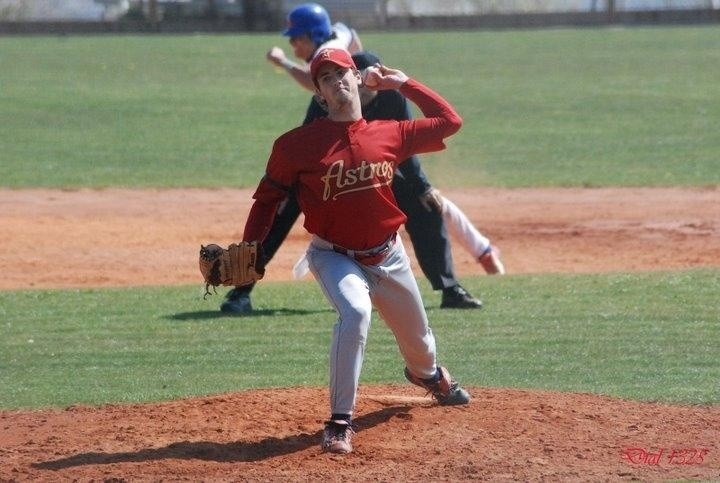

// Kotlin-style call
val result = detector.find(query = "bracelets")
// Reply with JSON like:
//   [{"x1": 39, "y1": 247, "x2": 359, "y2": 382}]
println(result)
[{"x1": 279, "y1": 56, "x2": 296, "y2": 73}]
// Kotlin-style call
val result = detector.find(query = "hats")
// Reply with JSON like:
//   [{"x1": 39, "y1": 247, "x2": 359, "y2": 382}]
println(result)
[{"x1": 309, "y1": 48, "x2": 356, "y2": 85}]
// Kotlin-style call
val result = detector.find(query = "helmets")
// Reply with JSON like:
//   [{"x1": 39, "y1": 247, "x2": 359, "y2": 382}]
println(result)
[{"x1": 283, "y1": 3, "x2": 333, "y2": 43}]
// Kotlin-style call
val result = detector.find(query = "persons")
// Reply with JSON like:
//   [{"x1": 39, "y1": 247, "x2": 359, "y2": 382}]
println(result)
[
  {"x1": 199, "y1": 43, "x2": 473, "y2": 455},
  {"x1": 264, "y1": 3, "x2": 506, "y2": 277},
  {"x1": 220, "y1": 49, "x2": 484, "y2": 315}
]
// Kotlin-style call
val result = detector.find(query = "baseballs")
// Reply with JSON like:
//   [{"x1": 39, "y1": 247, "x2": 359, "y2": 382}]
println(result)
[{"x1": 363, "y1": 66, "x2": 382, "y2": 88}]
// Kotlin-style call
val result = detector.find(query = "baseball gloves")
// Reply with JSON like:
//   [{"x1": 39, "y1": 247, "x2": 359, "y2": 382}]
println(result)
[{"x1": 199, "y1": 241, "x2": 266, "y2": 287}]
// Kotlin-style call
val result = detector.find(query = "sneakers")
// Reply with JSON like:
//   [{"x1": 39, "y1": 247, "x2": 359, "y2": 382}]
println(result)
[
  {"x1": 478, "y1": 246, "x2": 506, "y2": 275},
  {"x1": 438, "y1": 285, "x2": 484, "y2": 312},
  {"x1": 403, "y1": 366, "x2": 471, "y2": 407},
  {"x1": 219, "y1": 290, "x2": 253, "y2": 315},
  {"x1": 322, "y1": 418, "x2": 355, "y2": 459}
]
